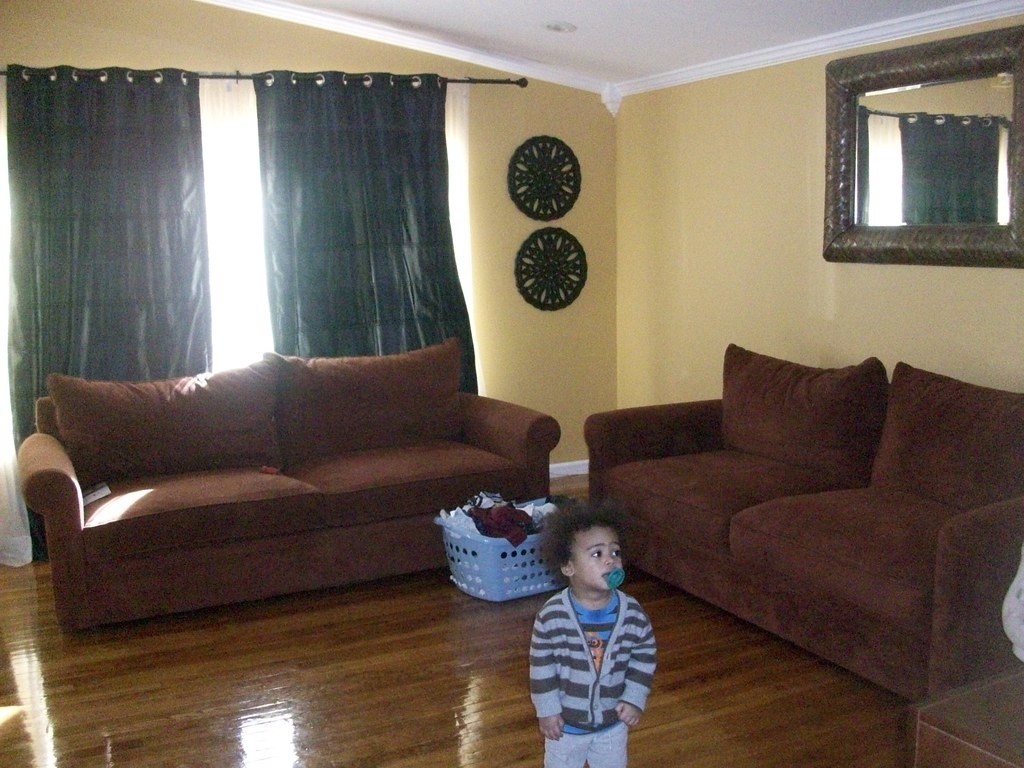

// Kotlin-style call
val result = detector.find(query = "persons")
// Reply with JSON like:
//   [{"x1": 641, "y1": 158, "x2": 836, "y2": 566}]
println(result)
[{"x1": 529, "y1": 498, "x2": 656, "y2": 768}]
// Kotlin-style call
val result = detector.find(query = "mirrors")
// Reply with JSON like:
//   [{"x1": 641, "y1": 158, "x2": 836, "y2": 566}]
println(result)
[{"x1": 823, "y1": 24, "x2": 1024, "y2": 269}]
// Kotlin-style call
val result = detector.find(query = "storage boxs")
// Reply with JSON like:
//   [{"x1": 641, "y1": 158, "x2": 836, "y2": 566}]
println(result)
[{"x1": 433, "y1": 498, "x2": 568, "y2": 602}]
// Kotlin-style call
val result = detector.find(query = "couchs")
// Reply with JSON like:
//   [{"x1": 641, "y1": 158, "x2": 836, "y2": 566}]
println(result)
[
  {"x1": 18, "y1": 339, "x2": 561, "y2": 633},
  {"x1": 584, "y1": 343, "x2": 1024, "y2": 704}
]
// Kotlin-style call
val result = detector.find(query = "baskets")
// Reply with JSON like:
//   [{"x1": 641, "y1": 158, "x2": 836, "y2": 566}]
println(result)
[{"x1": 433, "y1": 495, "x2": 588, "y2": 602}]
